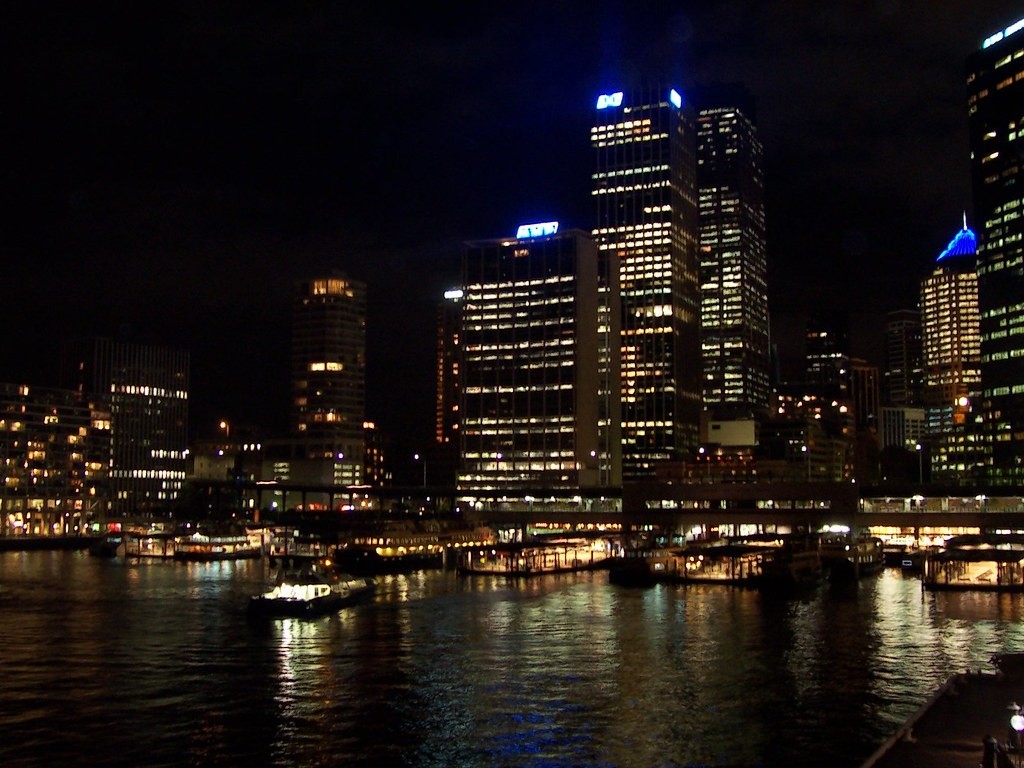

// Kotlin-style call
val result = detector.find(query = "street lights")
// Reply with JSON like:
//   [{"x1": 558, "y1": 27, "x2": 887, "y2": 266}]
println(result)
[{"x1": 915, "y1": 443, "x2": 922, "y2": 485}]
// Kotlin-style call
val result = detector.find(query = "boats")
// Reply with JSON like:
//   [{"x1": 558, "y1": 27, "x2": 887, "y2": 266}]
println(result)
[
  {"x1": 860, "y1": 651, "x2": 1024, "y2": 768},
  {"x1": 251, "y1": 578, "x2": 367, "y2": 619},
  {"x1": 91, "y1": 518, "x2": 887, "y2": 583},
  {"x1": 925, "y1": 542, "x2": 1024, "y2": 591}
]
